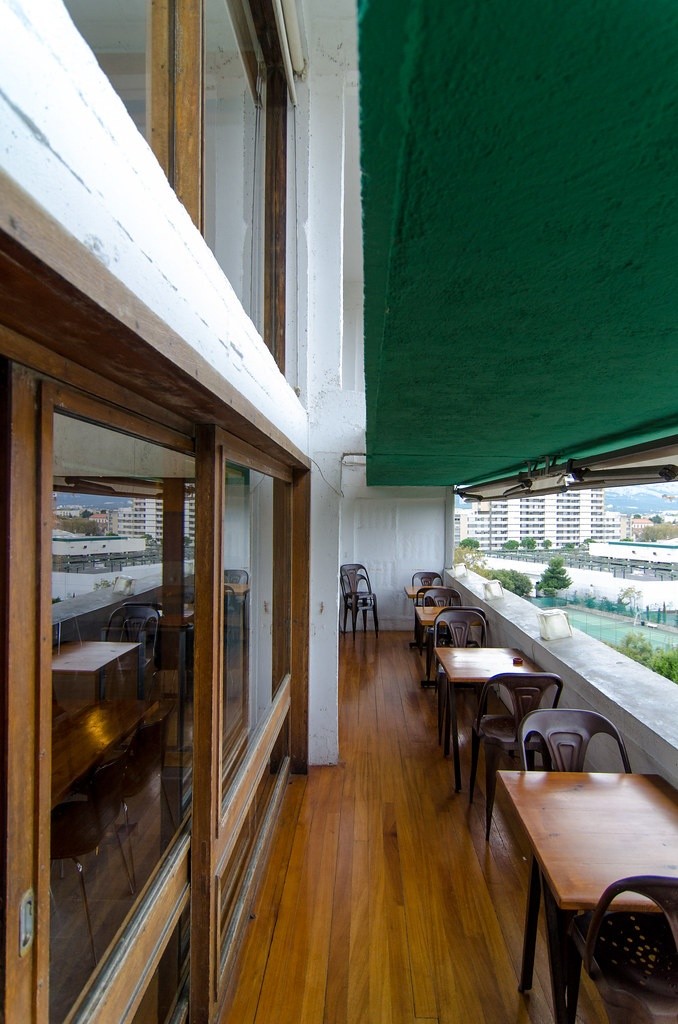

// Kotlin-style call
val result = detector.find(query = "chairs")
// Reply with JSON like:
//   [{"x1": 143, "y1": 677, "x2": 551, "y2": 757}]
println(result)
[
  {"x1": 469, "y1": 673, "x2": 564, "y2": 841},
  {"x1": 568, "y1": 875, "x2": 678, "y2": 1024},
  {"x1": 519, "y1": 709, "x2": 633, "y2": 1024},
  {"x1": 52, "y1": 703, "x2": 175, "y2": 969},
  {"x1": 434, "y1": 610, "x2": 488, "y2": 794},
  {"x1": 412, "y1": 572, "x2": 486, "y2": 689},
  {"x1": 341, "y1": 564, "x2": 379, "y2": 641},
  {"x1": 647, "y1": 623, "x2": 657, "y2": 629},
  {"x1": 51, "y1": 569, "x2": 250, "y2": 751}
]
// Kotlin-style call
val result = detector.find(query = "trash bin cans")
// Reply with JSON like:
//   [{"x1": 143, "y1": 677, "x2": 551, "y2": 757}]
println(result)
[{"x1": 536, "y1": 582, "x2": 544, "y2": 598}]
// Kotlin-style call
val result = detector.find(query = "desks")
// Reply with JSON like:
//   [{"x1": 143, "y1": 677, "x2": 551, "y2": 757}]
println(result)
[
  {"x1": 51, "y1": 699, "x2": 160, "y2": 811},
  {"x1": 223, "y1": 583, "x2": 250, "y2": 643},
  {"x1": 435, "y1": 648, "x2": 548, "y2": 755},
  {"x1": 52, "y1": 640, "x2": 142, "y2": 702},
  {"x1": 498, "y1": 770, "x2": 678, "y2": 1024},
  {"x1": 404, "y1": 586, "x2": 444, "y2": 648},
  {"x1": 415, "y1": 607, "x2": 481, "y2": 669},
  {"x1": 156, "y1": 602, "x2": 195, "y2": 622}
]
